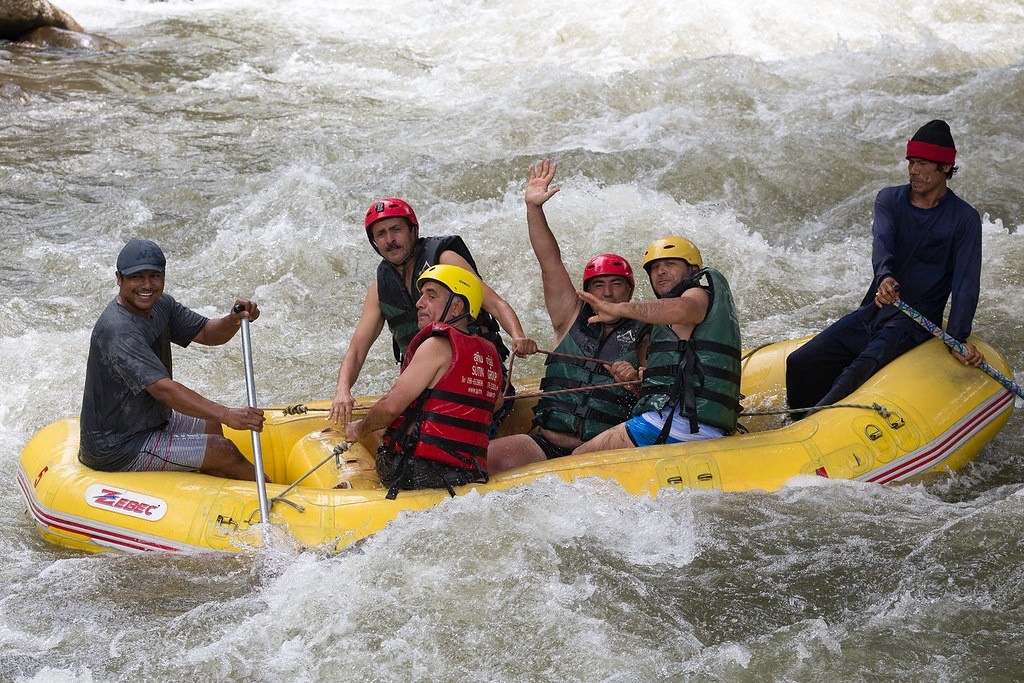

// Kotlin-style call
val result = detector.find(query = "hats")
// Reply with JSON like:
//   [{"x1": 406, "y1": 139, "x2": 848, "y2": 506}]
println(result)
[
  {"x1": 906, "y1": 119, "x2": 956, "y2": 164},
  {"x1": 117, "y1": 239, "x2": 166, "y2": 277}
]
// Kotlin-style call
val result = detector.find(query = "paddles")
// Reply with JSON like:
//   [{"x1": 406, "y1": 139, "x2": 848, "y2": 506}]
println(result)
[
  {"x1": 888, "y1": 291, "x2": 1023, "y2": 402},
  {"x1": 241, "y1": 320, "x2": 284, "y2": 568}
]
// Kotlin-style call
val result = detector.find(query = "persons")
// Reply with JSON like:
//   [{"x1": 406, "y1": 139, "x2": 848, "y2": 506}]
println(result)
[
  {"x1": 785, "y1": 119, "x2": 982, "y2": 424},
  {"x1": 328, "y1": 198, "x2": 537, "y2": 438},
  {"x1": 486, "y1": 158, "x2": 653, "y2": 476},
  {"x1": 77, "y1": 240, "x2": 273, "y2": 484},
  {"x1": 345, "y1": 265, "x2": 506, "y2": 502},
  {"x1": 572, "y1": 237, "x2": 742, "y2": 455}
]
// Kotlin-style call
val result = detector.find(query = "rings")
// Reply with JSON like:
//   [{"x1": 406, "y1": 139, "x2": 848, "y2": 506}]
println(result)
[{"x1": 876, "y1": 292, "x2": 880, "y2": 295}]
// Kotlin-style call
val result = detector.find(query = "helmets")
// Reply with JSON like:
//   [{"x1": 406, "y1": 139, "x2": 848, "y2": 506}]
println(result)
[
  {"x1": 365, "y1": 198, "x2": 418, "y2": 240},
  {"x1": 416, "y1": 264, "x2": 483, "y2": 322},
  {"x1": 582, "y1": 253, "x2": 635, "y2": 300},
  {"x1": 642, "y1": 236, "x2": 703, "y2": 270}
]
